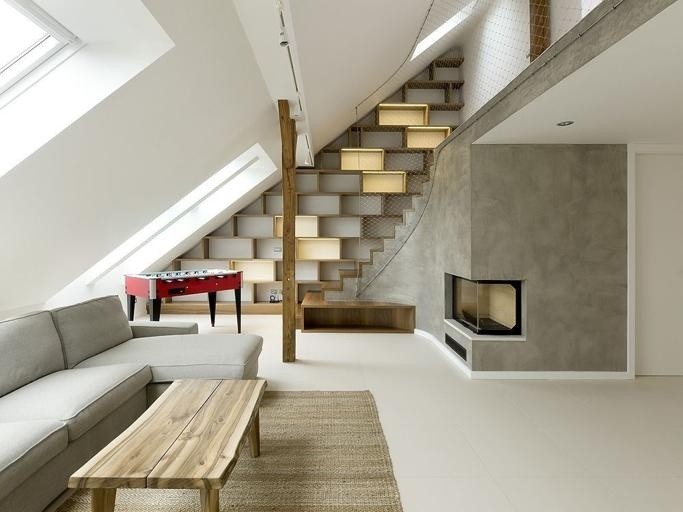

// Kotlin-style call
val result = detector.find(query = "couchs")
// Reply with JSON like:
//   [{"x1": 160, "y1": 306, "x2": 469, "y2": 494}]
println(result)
[
  {"x1": 50, "y1": 295, "x2": 264, "y2": 408},
  {"x1": 0, "y1": 310, "x2": 153, "y2": 512}
]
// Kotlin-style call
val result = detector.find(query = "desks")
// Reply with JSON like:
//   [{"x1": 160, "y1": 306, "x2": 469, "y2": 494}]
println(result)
[{"x1": 124, "y1": 268, "x2": 242, "y2": 334}]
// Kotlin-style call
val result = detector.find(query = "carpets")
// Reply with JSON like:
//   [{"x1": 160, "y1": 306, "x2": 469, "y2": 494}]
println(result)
[{"x1": 45, "y1": 390, "x2": 404, "y2": 512}]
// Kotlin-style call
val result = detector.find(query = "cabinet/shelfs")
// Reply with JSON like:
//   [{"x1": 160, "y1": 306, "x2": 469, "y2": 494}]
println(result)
[
  {"x1": 146, "y1": 56, "x2": 465, "y2": 314},
  {"x1": 300, "y1": 291, "x2": 416, "y2": 334}
]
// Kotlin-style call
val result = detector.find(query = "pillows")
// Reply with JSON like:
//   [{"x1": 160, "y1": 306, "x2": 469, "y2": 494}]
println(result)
[
  {"x1": 50, "y1": 295, "x2": 134, "y2": 370},
  {"x1": 0, "y1": 311, "x2": 66, "y2": 398}
]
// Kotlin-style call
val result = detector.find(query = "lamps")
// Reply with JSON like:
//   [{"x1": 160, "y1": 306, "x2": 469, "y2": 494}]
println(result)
[{"x1": 275, "y1": 1, "x2": 314, "y2": 168}]
